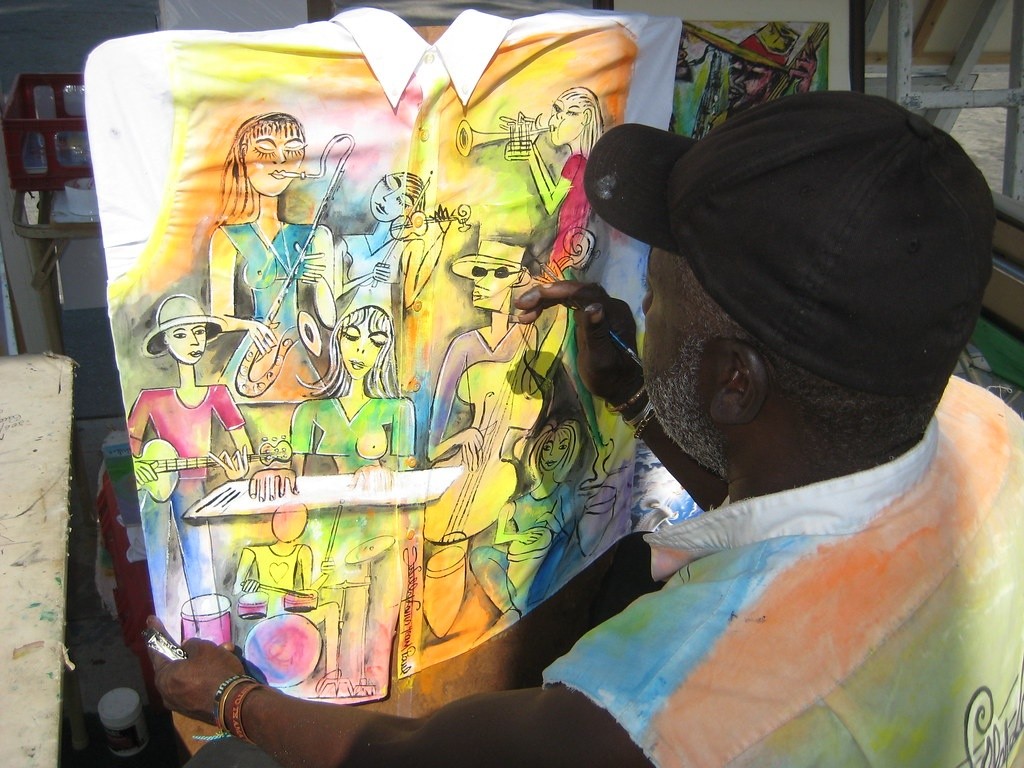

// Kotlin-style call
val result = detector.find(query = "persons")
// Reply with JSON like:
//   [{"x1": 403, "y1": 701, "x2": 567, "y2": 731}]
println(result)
[{"x1": 145, "y1": 91, "x2": 1024, "y2": 768}]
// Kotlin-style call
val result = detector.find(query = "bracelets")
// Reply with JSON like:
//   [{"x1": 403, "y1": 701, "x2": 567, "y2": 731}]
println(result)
[
  {"x1": 213, "y1": 673, "x2": 268, "y2": 747},
  {"x1": 605, "y1": 381, "x2": 656, "y2": 441}
]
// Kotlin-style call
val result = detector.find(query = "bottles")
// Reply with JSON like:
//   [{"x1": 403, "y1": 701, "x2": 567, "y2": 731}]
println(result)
[{"x1": 99, "y1": 687, "x2": 152, "y2": 759}]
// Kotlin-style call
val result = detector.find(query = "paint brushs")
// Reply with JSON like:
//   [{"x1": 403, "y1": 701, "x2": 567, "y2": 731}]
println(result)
[{"x1": 536, "y1": 258, "x2": 643, "y2": 370}]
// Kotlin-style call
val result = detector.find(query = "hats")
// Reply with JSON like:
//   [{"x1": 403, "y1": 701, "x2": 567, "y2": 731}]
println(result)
[{"x1": 581, "y1": 91, "x2": 998, "y2": 401}]
